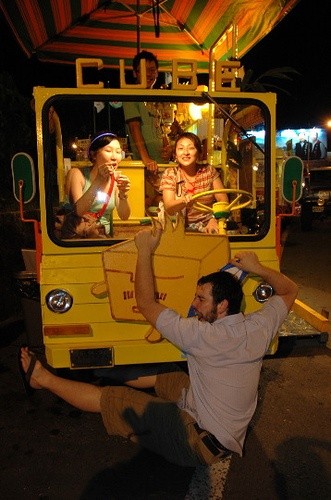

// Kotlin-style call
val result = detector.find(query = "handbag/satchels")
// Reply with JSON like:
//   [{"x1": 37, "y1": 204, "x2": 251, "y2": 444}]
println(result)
[{"x1": 53, "y1": 207, "x2": 112, "y2": 239}]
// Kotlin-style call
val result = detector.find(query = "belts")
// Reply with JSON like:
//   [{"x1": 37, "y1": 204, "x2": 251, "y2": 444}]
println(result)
[{"x1": 193, "y1": 423, "x2": 232, "y2": 458}]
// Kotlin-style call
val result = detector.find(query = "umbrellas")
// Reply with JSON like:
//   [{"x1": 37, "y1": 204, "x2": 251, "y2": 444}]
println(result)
[{"x1": 0, "y1": 0, "x2": 298, "y2": 78}]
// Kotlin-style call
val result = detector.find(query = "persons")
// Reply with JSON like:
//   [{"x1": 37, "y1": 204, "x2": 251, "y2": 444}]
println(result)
[
  {"x1": 15, "y1": 227, "x2": 300, "y2": 471},
  {"x1": 295, "y1": 133, "x2": 312, "y2": 160},
  {"x1": 122, "y1": 51, "x2": 189, "y2": 209},
  {"x1": 62, "y1": 131, "x2": 132, "y2": 238},
  {"x1": 158, "y1": 132, "x2": 230, "y2": 235},
  {"x1": 309, "y1": 132, "x2": 326, "y2": 160}
]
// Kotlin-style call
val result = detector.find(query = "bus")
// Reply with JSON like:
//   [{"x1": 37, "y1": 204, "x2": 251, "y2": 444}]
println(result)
[{"x1": 11, "y1": 84, "x2": 304, "y2": 373}]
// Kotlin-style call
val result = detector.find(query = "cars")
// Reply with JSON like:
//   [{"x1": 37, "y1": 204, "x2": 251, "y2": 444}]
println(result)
[{"x1": 302, "y1": 166, "x2": 331, "y2": 214}]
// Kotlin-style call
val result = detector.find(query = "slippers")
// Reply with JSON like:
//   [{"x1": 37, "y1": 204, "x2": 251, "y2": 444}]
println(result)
[{"x1": 17, "y1": 343, "x2": 43, "y2": 397}]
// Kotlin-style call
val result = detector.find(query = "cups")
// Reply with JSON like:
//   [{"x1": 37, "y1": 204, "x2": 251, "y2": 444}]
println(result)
[{"x1": 113, "y1": 171, "x2": 121, "y2": 181}]
